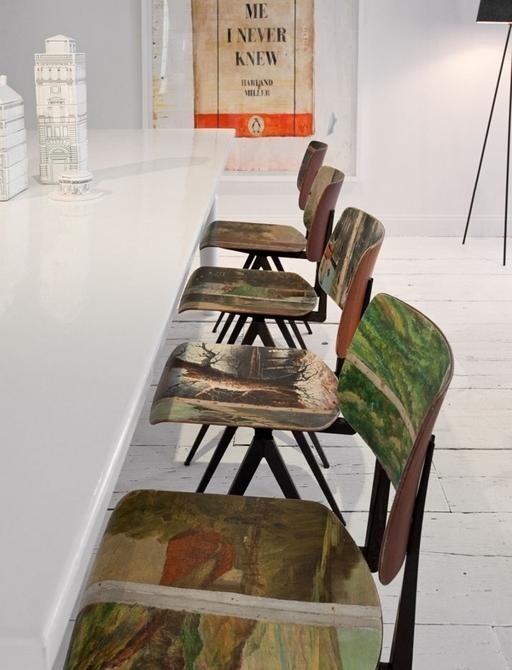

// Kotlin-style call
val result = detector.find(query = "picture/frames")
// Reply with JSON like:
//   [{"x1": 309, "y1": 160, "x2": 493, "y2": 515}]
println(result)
[{"x1": 142, "y1": 0, "x2": 364, "y2": 183}]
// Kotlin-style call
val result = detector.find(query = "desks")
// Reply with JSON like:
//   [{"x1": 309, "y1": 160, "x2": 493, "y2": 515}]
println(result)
[{"x1": 0, "y1": 129, "x2": 236, "y2": 670}]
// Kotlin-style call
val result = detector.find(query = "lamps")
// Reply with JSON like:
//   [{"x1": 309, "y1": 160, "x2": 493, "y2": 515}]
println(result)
[{"x1": 462, "y1": 0, "x2": 512, "y2": 266}]
